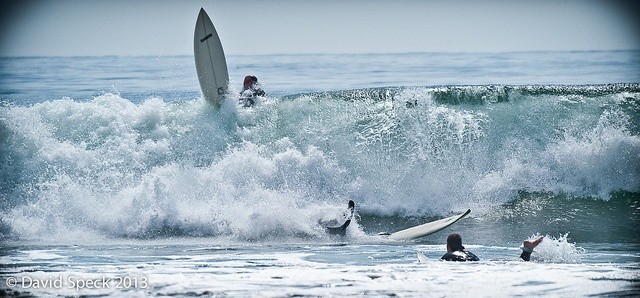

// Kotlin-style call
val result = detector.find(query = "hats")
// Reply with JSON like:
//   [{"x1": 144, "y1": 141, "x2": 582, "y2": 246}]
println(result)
[{"x1": 447, "y1": 234, "x2": 465, "y2": 249}]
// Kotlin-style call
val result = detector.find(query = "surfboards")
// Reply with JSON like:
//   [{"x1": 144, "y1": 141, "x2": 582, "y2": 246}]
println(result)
[
  {"x1": 194, "y1": 7, "x2": 230, "y2": 105},
  {"x1": 417, "y1": 251, "x2": 435, "y2": 264},
  {"x1": 371, "y1": 209, "x2": 471, "y2": 241}
]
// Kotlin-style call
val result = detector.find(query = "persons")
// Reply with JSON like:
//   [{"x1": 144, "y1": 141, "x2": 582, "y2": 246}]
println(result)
[
  {"x1": 439, "y1": 233, "x2": 544, "y2": 263},
  {"x1": 239, "y1": 75, "x2": 266, "y2": 108}
]
